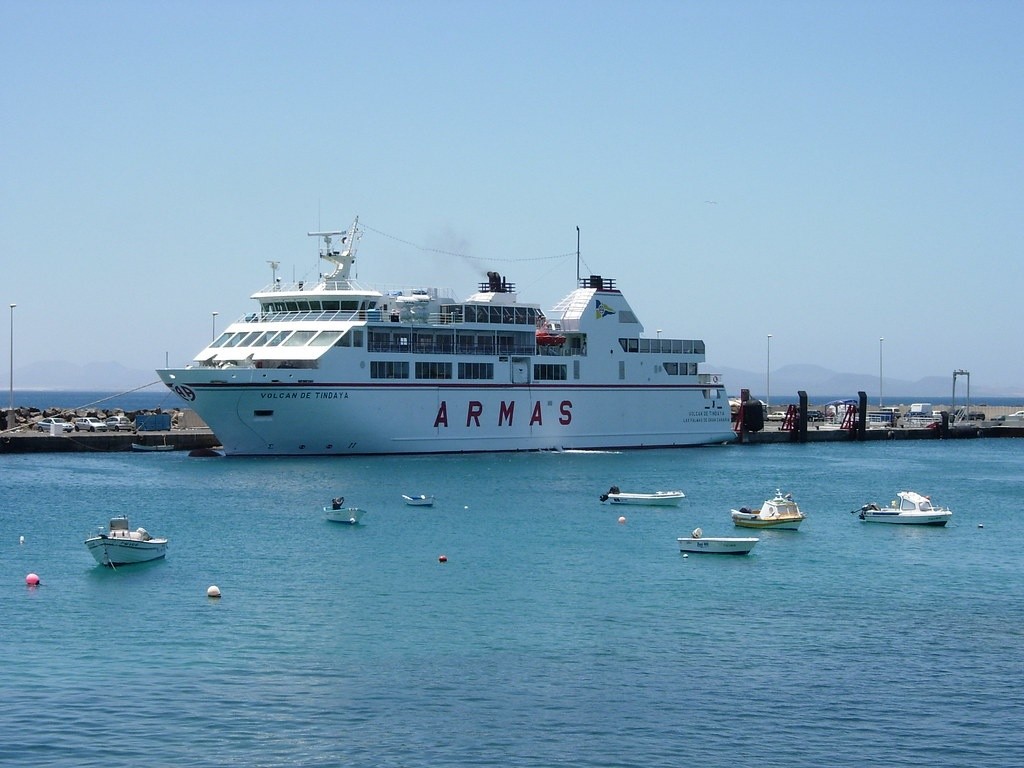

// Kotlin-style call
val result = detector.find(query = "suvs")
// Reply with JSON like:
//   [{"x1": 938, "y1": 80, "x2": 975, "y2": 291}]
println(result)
[{"x1": 807, "y1": 411, "x2": 821, "y2": 422}]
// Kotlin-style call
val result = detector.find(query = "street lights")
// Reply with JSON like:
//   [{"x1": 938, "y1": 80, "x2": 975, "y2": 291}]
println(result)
[
  {"x1": 880, "y1": 338, "x2": 884, "y2": 409},
  {"x1": 768, "y1": 334, "x2": 772, "y2": 408},
  {"x1": 10, "y1": 303, "x2": 19, "y2": 409}
]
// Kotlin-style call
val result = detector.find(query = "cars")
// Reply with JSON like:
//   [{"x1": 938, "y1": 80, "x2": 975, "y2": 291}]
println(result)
[
  {"x1": 75, "y1": 415, "x2": 132, "y2": 433},
  {"x1": 1009, "y1": 411, "x2": 1024, "y2": 418},
  {"x1": 990, "y1": 415, "x2": 1005, "y2": 421},
  {"x1": 767, "y1": 411, "x2": 791, "y2": 422},
  {"x1": 969, "y1": 411, "x2": 985, "y2": 420},
  {"x1": 37, "y1": 418, "x2": 74, "y2": 432}
]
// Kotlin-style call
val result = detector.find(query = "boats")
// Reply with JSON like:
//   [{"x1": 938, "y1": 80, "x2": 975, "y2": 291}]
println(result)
[
  {"x1": 535, "y1": 330, "x2": 568, "y2": 346},
  {"x1": 599, "y1": 486, "x2": 685, "y2": 506},
  {"x1": 850, "y1": 491, "x2": 951, "y2": 526},
  {"x1": 132, "y1": 441, "x2": 175, "y2": 452},
  {"x1": 402, "y1": 494, "x2": 435, "y2": 506},
  {"x1": 323, "y1": 505, "x2": 367, "y2": 524},
  {"x1": 731, "y1": 487, "x2": 806, "y2": 530},
  {"x1": 677, "y1": 528, "x2": 759, "y2": 554},
  {"x1": 155, "y1": 215, "x2": 738, "y2": 454},
  {"x1": 85, "y1": 517, "x2": 168, "y2": 566}
]
